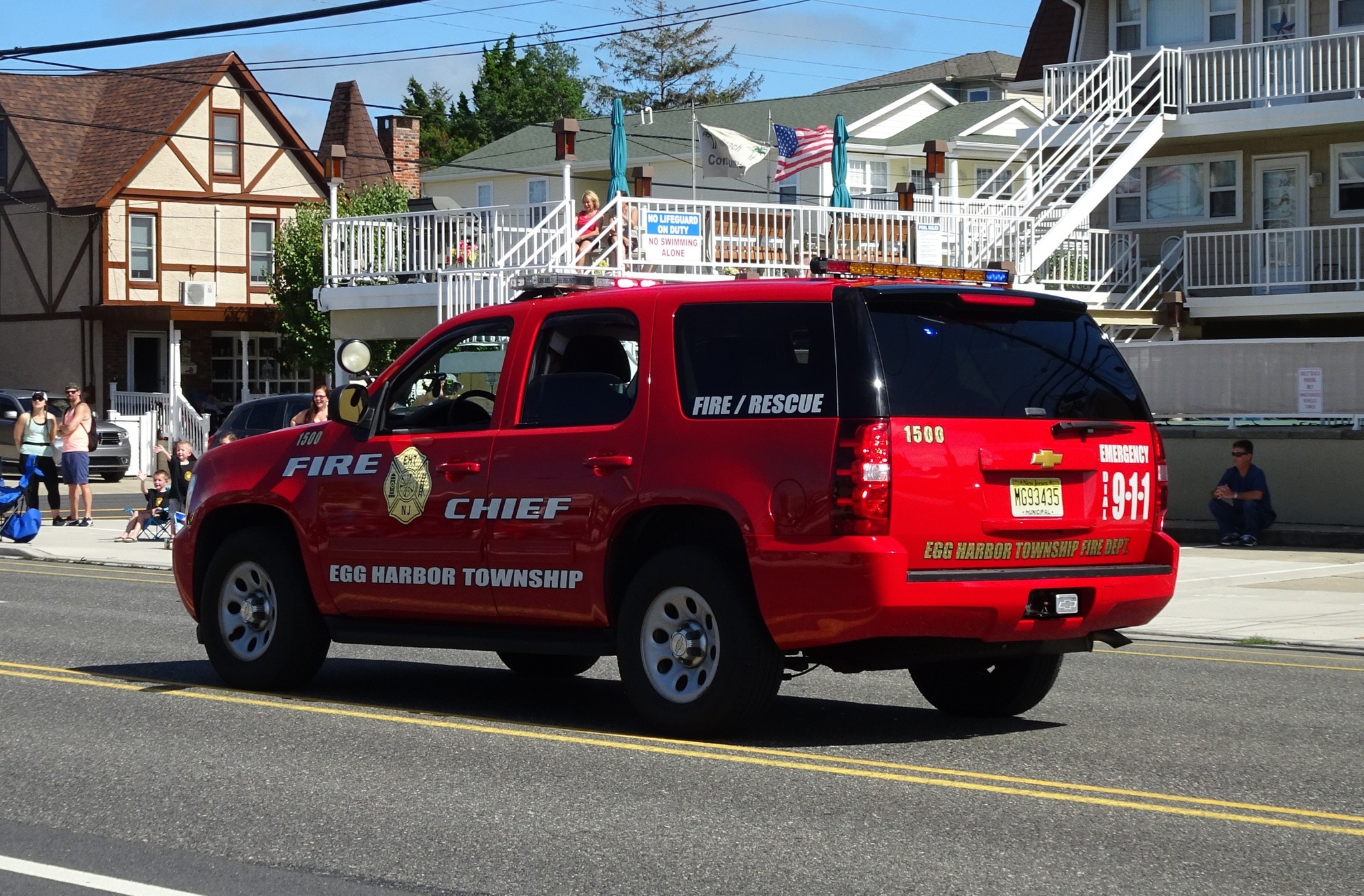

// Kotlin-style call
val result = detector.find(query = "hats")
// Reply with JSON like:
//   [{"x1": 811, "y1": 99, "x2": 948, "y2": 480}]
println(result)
[
  {"x1": 65, "y1": 382, "x2": 81, "y2": 390},
  {"x1": 32, "y1": 391, "x2": 48, "y2": 401}
]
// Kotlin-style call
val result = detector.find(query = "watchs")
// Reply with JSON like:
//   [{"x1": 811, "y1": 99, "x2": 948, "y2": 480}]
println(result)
[{"x1": 1232, "y1": 492, "x2": 1238, "y2": 498}]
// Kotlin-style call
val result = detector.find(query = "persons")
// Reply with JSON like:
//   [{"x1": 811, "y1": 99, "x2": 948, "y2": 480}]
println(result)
[
  {"x1": 574, "y1": 190, "x2": 639, "y2": 274},
  {"x1": 115, "y1": 441, "x2": 196, "y2": 550},
  {"x1": 216, "y1": 433, "x2": 240, "y2": 446},
  {"x1": 14, "y1": 381, "x2": 99, "y2": 528},
  {"x1": 289, "y1": 385, "x2": 330, "y2": 428},
  {"x1": 1209, "y1": 438, "x2": 1277, "y2": 547}
]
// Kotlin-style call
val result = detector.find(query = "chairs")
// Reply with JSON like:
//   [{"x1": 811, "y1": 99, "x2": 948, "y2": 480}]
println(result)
[
  {"x1": 1080, "y1": 71, "x2": 1123, "y2": 113},
  {"x1": 1090, "y1": 238, "x2": 1129, "y2": 291},
  {"x1": 122, "y1": 486, "x2": 185, "y2": 540},
  {"x1": 1132, "y1": 66, "x2": 1177, "y2": 114},
  {"x1": 1139, "y1": 236, "x2": 1184, "y2": 295},
  {"x1": 540, "y1": 333, "x2": 632, "y2": 423}
]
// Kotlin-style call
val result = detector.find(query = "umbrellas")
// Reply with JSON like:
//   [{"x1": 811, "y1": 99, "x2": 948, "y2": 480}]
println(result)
[
  {"x1": 827, "y1": 113, "x2": 853, "y2": 258},
  {"x1": 606, "y1": 98, "x2": 630, "y2": 205}
]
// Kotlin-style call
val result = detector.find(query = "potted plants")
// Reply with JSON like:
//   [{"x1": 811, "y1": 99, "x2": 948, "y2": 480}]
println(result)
[{"x1": 793, "y1": 247, "x2": 817, "y2": 278}]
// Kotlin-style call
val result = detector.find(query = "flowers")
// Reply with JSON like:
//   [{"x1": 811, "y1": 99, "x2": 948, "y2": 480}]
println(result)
[{"x1": 445, "y1": 239, "x2": 478, "y2": 266}]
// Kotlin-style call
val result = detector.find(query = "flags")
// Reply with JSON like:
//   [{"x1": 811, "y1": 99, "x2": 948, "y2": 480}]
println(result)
[
  {"x1": 772, "y1": 122, "x2": 836, "y2": 182},
  {"x1": 696, "y1": 119, "x2": 773, "y2": 178}
]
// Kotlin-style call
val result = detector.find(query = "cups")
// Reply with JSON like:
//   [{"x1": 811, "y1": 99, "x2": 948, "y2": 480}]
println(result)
[{"x1": 164, "y1": 538, "x2": 170, "y2": 549}]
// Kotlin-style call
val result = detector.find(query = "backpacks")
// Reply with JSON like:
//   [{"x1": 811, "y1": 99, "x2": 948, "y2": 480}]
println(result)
[{"x1": 63, "y1": 401, "x2": 99, "y2": 452}]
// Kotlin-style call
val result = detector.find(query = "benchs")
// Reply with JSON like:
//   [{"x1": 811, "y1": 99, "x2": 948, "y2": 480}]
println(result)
[
  {"x1": 583, "y1": 207, "x2": 649, "y2": 270},
  {"x1": 805, "y1": 216, "x2": 915, "y2": 265},
  {"x1": 706, "y1": 209, "x2": 796, "y2": 261}
]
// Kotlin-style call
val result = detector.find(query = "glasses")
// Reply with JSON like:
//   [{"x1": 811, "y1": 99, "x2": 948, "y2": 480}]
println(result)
[
  {"x1": 1232, "y1": 452, "x2": 1247, "y2": 457},
  {"x1": 313, "y1": 395, "x2": 326, "y2": 399},
  {"x1": 65, "y1": 389, "x2": 78, "y2": 394},
  {"x1": 32, "y1": 397, "x2": 44, "y2": 402}
]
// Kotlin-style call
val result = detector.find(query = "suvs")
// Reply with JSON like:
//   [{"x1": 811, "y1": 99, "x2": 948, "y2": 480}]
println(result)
[
  {"x1": 0, "y1": 388, "x2": 131, "y2": 482},
  {"x1": 169, "y1": 255, "x2": 1182, "y2": 738},
  {"x1": 206, "y1": 393, "x2": 315, "y2": 450}
]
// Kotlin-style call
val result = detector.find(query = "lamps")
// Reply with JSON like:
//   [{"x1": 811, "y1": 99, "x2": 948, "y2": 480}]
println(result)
[
  {"x1": 551, "y1": 117, "x2": 581, "y2": 161},
  {"x1": 631, "y1": 166, "x2": 654, "y2": 197},
  {"x1": 895, "y1": 182, "x2": 916, "y2": 211},
  {"x1": 1309, "y1": 171, "x2": 1323, "y2": 189},
  {"x1": 322, "y1": 144, "x2": 348, "y2": 184},
  {"x1": 922, "y1": 140, "x2": 948, "y2": 179}
]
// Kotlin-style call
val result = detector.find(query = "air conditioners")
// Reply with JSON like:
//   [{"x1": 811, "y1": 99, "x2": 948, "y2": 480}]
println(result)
[{"x1": 181, "y1": 281, "x2": 216, "y2": 307}]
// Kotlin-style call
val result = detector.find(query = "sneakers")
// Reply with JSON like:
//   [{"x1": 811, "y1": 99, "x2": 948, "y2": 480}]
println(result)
[
  {"x1": 1220, "y1": 534, "x2": 1240, "y2": 545},
  {"x1": 66, "y1": 515, "x2": 80, "y2": 526},
  {"x1": 52, "y1": 515, "x2": 67, "y2": 526},
  {"x1": 1240, "y1": 534, "x2": 1258, "y2": 546},
  {"x1": 78, "y1": 516, "x2": 94, "y2": 526}
]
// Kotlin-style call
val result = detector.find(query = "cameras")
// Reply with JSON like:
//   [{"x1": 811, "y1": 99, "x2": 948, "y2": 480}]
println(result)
[{"x1": 443, "y1": 379, "x2": 455, "y2": 395}]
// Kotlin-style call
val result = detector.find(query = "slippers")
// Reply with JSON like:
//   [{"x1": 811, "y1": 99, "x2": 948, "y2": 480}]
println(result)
[
  {"x1": 114, "y1": 537, "x2": 125, "y2": 542},
  {"x1": 123, "y1": 537, "x2": 139, "y2": 543}
]
